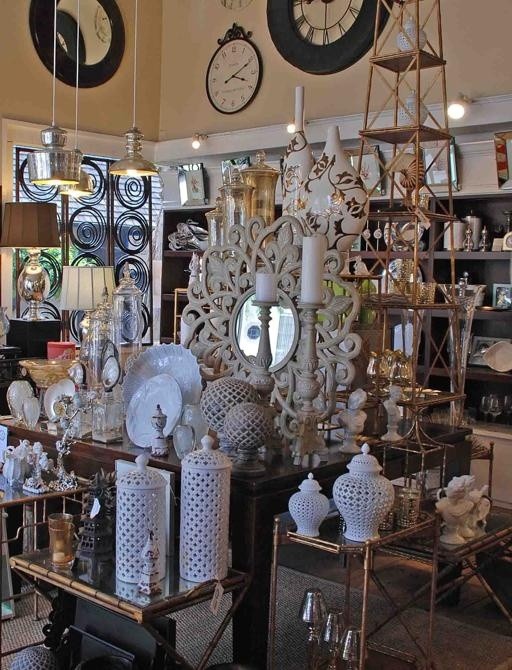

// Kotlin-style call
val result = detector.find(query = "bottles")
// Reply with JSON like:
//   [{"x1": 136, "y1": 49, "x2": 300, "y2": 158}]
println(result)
[
  {"x1": 84, "y1": 301, "x2": 111, "y2": 395},
  {"x1": 218, "y1": 166, "x2": 255, "y2": 252},
  {"x1": 239, "y1": 150, "x2": 282, "y2": 252},
  {"x1": 441, "y1": 213, "x2": 468, "y2": 252},
  {"x1": 478, "y1": 224, "x2": 491, "y2": 252},
  {"x1": 462, "y1": 224, "x2": 474, "y2": 252},
  {"x1": 91, "y1": 388, "x2": 124, "y2": 444},
  {"x1": 205, "y1": 196, "x2": 225, "y2": 260},
  {"x1": 111, "y1": 262, "x2": 145, "y2": 374},
  {"x1": 461, "y1": 208, "x2": 484, "y2": 251}
]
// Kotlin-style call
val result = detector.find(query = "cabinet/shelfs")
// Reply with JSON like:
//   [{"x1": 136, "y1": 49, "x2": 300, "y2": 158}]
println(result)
[
  {"x1": 159, "y1": 202, "x2": 281, "y2": 343},
  {"x1": 348, "y1": 0, "x2": 466, "y2": 436},
  {"x1": 328, "y1": 190, "x2": 512, "y2": 509}
]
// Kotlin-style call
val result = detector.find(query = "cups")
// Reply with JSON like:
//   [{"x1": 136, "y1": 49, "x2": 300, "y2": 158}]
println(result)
[
  {"x1": 173, "y1": 424, "x2": 196, "y2": 461},
  {"x1": 336, "y1": 485, "x2": 423, "y2": 536},
  {"x1": 48, "y1": 512, "x2": 74, "y2": 554},
  {"x1": 412, "y1": 192, "x2": 430, "y2": 210},
  {"x1": 47, "y1": 521, "x2": 76, "y2": 569},
  {"x1": 5, "y1": 380, "x2": 36, "y2": 421},
  {"x1": 22, "y1": 397, "x2": 41, "y2": 430},
  {"x1": 181, "y1": 403, "x2": 210, "y2": 449},
  {"x1": 391, "y1": 279, "x2": 436, "y2": 304}
]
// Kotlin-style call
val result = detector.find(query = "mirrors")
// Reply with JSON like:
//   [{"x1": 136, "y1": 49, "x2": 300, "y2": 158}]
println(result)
[{"x1": 230, "y1": 287, "x2": 301, "y2": 371}]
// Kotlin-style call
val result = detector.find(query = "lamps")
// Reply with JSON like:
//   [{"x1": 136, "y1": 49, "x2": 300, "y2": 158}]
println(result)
[
  {"x1": 28, "y1": 0, "x2": 160, "y2": 198},
  {"x1": 1, "y1": 202, "x2": 62, "y2": 319}
]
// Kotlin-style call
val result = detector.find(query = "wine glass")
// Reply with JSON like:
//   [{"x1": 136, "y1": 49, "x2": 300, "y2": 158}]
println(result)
[
  {"x1": 479, "y1": 393, "x2": 512, "y2": 428},
  {"x1": 367, "y1": 347, "x2": 415, "y2": 402}
]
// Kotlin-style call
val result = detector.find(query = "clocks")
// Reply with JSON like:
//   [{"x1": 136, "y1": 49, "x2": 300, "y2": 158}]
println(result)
[{"x1": 205, "y1": 22, "x2": 262, "y2": 115}]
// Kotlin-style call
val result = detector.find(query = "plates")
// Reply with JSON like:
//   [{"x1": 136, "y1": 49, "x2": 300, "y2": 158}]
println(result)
[
  {"x1": 381, "y1": 259, "x2": 423, "y2": 295},
  {"x1": 124, "y1": 373, "x2": 183, "y2": 449},
  {"x1": 121, "y1": 341, "x2": 204, "y2": 437},
  {"x1": 382, "y1": 221, "x2": 425, "y2": 252},
  {"x1": 44, "y1": 378, "x2": 78, "y2": 423},
  {"x1": 482, "y1": 340, "x2": 512, "y2": 373}
]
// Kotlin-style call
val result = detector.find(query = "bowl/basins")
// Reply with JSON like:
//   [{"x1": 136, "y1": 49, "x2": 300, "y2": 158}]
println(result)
[{"x1": 17, "y1": 359, "x2": 85, "y2": 389}]
